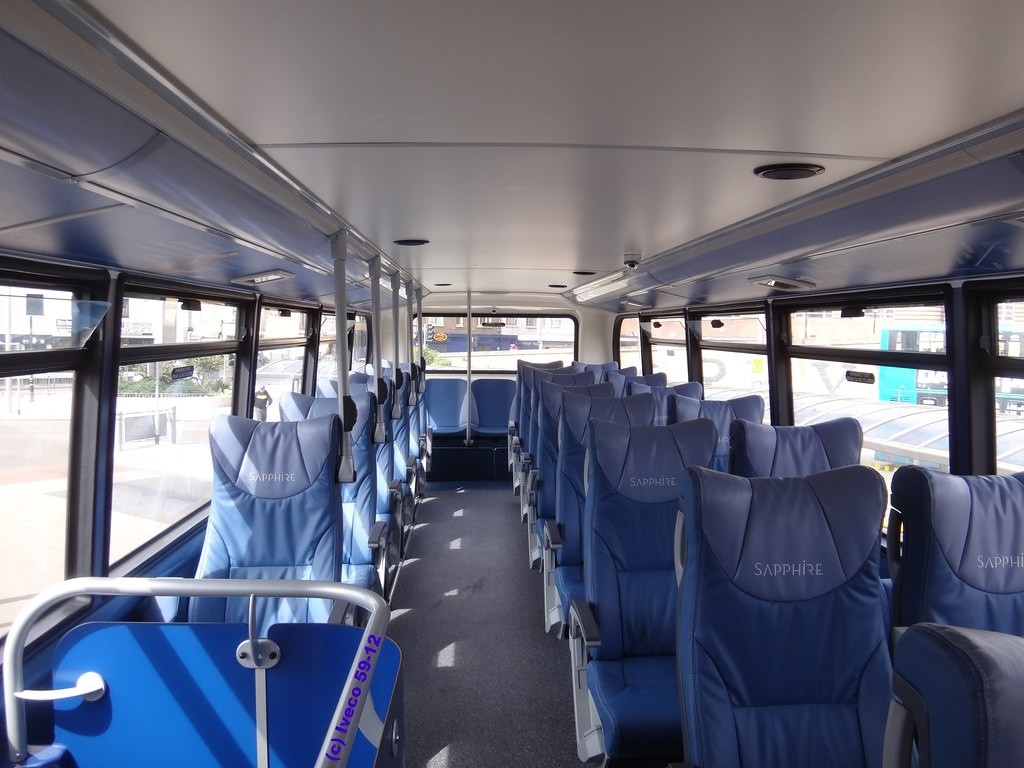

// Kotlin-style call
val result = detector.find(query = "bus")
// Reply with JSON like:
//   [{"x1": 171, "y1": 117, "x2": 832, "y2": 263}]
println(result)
[{"x1": 874, "y1": 325, "x2": 1024, "y2": 474}]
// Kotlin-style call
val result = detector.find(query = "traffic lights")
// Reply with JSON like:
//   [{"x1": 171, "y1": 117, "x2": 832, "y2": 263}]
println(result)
[{"x1": 425, "y1": 324, "x2": 434, "y2": 342}]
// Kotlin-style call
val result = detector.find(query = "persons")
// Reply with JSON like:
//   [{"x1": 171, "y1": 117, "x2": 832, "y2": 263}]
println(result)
[{"x1": 253, "y1": 383, "x2": 273, "y2": 422}]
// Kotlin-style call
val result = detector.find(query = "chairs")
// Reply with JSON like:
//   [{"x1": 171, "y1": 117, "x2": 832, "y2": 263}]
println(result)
[
  {"x1": 569, "y1": 418, "x2": 719, "y2": 767},
  {"x1": 729, "y1": 416, "x2": 863, "y2": 475},
  {"x1": 279, "y1": 391, "x2": 382, "y2": 596},
  {"x1": 471, "y1": 378, "x2": 517, "y2": 434},
  {"x1": 897, "y1": 623, "x2": 1024, "y2": 767},
  {"x1": 188, "y1": 413, "x2": 342, "y2": 623},
  {"x1": 887, "y1": 465, "x2": 1024, "y2": 636},
  {"x1": 508, "y1": 358, "x2": 765, "y2": 639},
  {"x1": 674, "y1": 465, "x2": 918, "y2": 767},
  {"x1": 318, "y1": 357, "x2": 427, "y2": 538},
  {"x1": 424, "y1": 377, "x2": 467, "y2": 433}
]
